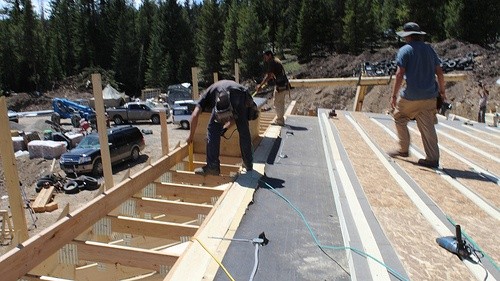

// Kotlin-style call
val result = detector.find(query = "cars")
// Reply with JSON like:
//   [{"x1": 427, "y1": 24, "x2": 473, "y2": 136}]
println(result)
[
  {"x1": 8, "y1": 110, "x2": 19, "y2": 123},
  {"x1": 172, "y1": 99, "x2": 197, "y2": 129}
]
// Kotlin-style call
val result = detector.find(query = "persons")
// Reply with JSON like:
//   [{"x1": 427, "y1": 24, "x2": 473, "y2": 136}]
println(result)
[
  {"x1": 477, "y1": 85, "x2": 489, "y2": 123},
  {"x1": 387, "y1": 21, "x2": 446, "y2": 169},
  {"x1": 184, "y1": 80, "x2": 260, "y2": 176},
  {"x1": 256, "y1": 50, "x2": 292, "y2": 126}
]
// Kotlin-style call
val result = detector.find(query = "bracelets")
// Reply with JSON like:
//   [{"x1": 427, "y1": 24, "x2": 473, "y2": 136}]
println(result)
[{"x1": 391, "y1": 96, "x2": 397, "y2": 98}]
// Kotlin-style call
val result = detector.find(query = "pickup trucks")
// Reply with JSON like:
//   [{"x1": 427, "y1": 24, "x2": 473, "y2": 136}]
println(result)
[{"x1": 107, "y1": 102, "x2": 161, "y2": 125}]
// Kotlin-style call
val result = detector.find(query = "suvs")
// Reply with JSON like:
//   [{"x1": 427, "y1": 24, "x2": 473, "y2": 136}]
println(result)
[{"x1": 59, "y1": 125, "x2": 146, "y2": 179}]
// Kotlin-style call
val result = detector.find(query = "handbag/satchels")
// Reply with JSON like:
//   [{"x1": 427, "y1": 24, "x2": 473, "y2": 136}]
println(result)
[{"x1": 275, "y1": 75, "x2": 290, "y2": 91}]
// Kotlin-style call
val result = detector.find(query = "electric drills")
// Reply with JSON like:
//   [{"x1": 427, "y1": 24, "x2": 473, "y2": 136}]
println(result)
[
  {"x1": 436, "y1": 224, "x2": 482, "y2": 266},
  {"x1": 435, "y1": 93, "x2": 452, "y2": 111}
]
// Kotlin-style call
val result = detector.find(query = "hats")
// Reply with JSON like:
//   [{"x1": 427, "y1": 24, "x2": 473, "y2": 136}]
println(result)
[{"x1": 396, "y1": 22, "x2": 427, "y2": 38}]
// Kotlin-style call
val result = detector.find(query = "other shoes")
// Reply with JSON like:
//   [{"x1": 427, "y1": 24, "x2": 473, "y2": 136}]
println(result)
[
  {"x1": 194, "y1": 166, "x2": 220, "y2": 176},
  {"x1": 387, "y1": 150, "x2": 409, "y2": 157},
  {"x1": 240, "y1": 164, "x2": 253, "y2": 174},
  {"x1": 418, "y1": 159, "x2": 439, "y2": 168},
  {"x1": 271, "y1": 118, "x2": 284, "y2": 126}
]
